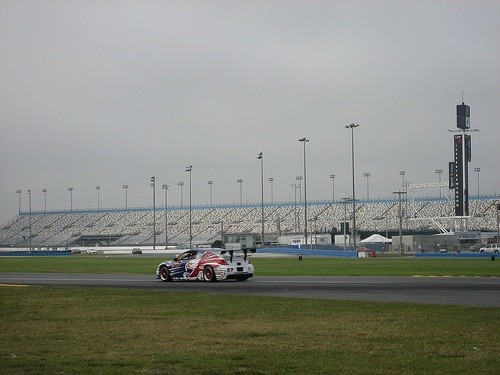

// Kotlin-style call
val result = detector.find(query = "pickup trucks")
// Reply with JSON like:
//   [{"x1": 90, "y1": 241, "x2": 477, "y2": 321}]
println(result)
[
  {"x1": 71, "y1": 249, "x2": 81, "y2": 254},
  {"x1": 440, "y1": 247, "x2": 447, "y2": 253}
]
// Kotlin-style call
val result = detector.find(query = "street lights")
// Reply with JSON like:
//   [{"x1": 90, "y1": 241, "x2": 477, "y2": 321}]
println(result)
[
  {"x1": 237, "y1": 179, "x2": 244, "y2": 207},
  {"x1": 330, "y1": 174, "x2": 336, "y2": 202},
  {"x1": 436, "y1": 169, "x2": 443, "y2": 198},
  {"x1": 474, "y1": 167, "x2": 481, "y2": 199},
  {"x1": 218, "y1": 221, "x2": 225, "y2": 248},
  {"x1": 68, "y1": 187, "x2": 73, "y2": 211},
  {"x1": 123, "y1": 185, "x2": 128, "y2": 210},
  {"x1": 208, "y1": 180, "x2": 212, "y2": 206},
  {"x1": 96, "y1": 186, "x2": 100, "y2": 210},
  {"x1": 257, "y1": 151, "x2": 268, "y2": 247},
  {"x1": 185, "y1": 165, "x2": 193, "y2": 247},
  {"x1": 268, "y1": 178, "x2": 274, "y2": 204},
  {"x1": 151, "y1": 176, "x2": 184, "y2": 250},
  {"x1": 345, "y1": 123, "x2": 359, "y2": 250},
  {"x1": 399, "y1": 170, "x2": 413, "y2": 218},
  {"x1": 290, "y1": 176, "x2": 304, "y2": 234},
  {"x1": 16, "y1": 189, "x2": 46, "y2": 251},
  {"x1": 364, "y1": 172, "x2": 371, "y2": 202},
  {"x1": 298, "y1": 137, "x2": 309, "y2": 249}
]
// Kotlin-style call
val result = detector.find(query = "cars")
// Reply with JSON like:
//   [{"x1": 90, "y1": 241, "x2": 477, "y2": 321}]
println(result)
[
  {"x1": 479, "y1": 242, "x2": 500, "y2": 254},
  {"x1": 469, "y1": 244, "x2": 484, "y2": 252},
  {"x1": 132, "y1": 248, "x2": 142, "y2": 255},
  {"x1": 86, "y1": 249, "x2": 98, "y2": 255}
]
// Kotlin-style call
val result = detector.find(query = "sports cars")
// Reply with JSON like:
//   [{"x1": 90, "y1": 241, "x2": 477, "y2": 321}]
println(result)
[{"x1": 154, "y1": 247, "x2": 254, "y2": 283}]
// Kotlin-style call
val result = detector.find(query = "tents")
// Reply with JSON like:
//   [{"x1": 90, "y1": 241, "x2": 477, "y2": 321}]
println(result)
[{"x1": 360, "y1": 234, "x2": 393, "y2": 243}]
